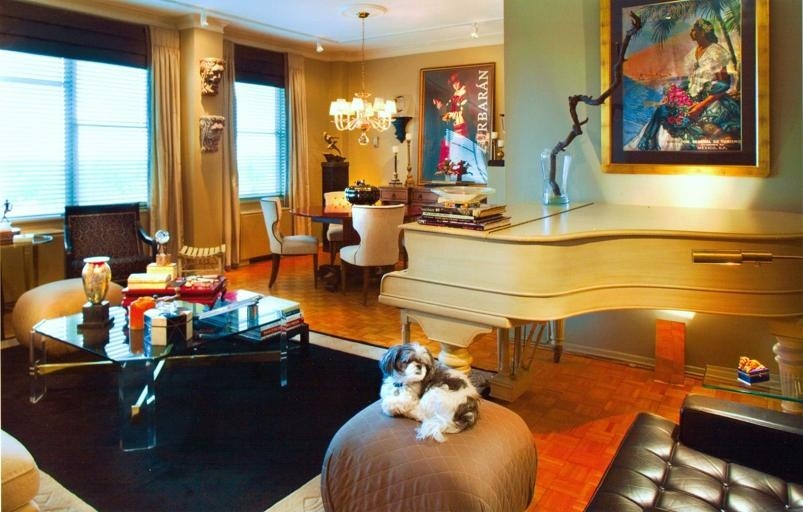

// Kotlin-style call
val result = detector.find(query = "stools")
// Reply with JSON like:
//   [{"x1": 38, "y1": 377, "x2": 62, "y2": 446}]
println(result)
[
  {"x1": 12, "y1": 277, "x2": 127, "y2": 358},
  {"x1": 178, "y1": 243, "x2": 227, "y2": 276},
  {"x1": 320, "y1": 395, "x2": 537, "y2": 512}
]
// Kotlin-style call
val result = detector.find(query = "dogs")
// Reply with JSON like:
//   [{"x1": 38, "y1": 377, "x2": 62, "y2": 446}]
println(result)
[{"x1": 378, "y1": 343, "x2": 482, "y2": 443}]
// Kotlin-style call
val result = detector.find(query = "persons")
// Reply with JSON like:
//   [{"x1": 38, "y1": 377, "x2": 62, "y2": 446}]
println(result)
[
  {"x1": 432, "y1": 72, "x2": 479, "y2": 183},
  {"x1": 199, "y1": 115, "x2": 227, "y2": 153},
  {"x1": 623, "y1": 19, "x2": 741, "y2": 152},
  {"x1": 201, "y1": 57, "x2": 227, "y2": 96}
]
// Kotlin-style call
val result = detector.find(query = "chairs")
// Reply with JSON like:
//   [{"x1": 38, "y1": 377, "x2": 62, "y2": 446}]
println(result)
[
  {"x1": 324, "y1": 190, "x2": 382, "y2": 267},
  {"x1": 62, "y1": 202, "x2": 157, "y2": 288},
  {"x1": 339, "y1": 204, "x2": 405, "y2": 302},
  {"x1": 261, "y1": 197, "x2": 319, "y2": 289}
]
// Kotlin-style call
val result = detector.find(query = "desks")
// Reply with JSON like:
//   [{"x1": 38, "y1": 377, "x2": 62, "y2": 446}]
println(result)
[
  {"x1": 0, "y1": 232, "x2": 54, "y2": 340},
  {"x1": 288, "y1": 205, "x2": 422, "y2": 284}
]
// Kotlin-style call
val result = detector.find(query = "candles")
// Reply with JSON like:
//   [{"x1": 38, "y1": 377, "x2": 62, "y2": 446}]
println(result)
[
  {"x1": 392, "y1": 146, "x2": 398, "y2": 152},
  {"x1": 406, "y1": 133, "x2": 411, "y2": 140}
]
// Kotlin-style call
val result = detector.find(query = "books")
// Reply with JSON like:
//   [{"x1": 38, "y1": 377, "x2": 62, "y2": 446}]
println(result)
[{"x1": 417, "y1": 201, "x2": 512, "y2": 230}]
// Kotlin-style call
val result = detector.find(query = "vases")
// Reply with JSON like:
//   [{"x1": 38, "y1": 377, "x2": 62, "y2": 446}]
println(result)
[{"x1": 540, "y1": 149, "x2": 574, "y2": 206}]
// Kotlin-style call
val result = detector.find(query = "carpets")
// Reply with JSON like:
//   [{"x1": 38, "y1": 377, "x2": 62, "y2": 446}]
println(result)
[{"x1": 1, "y1": 338, "x2": 382, "y2": 509}]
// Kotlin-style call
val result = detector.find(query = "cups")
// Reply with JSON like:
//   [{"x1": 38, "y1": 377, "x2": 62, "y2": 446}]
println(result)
[{"x1": 183, "y1": 275, "x2": 219, "y2": 288}]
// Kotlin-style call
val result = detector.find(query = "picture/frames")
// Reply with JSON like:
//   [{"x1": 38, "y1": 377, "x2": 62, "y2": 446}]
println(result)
[
  {"x1": 598, "y1": 0, "x2": 769, "y2": 177},
  {"x1": 416, "y1": 62, "x2": 493, "y2": 187}
]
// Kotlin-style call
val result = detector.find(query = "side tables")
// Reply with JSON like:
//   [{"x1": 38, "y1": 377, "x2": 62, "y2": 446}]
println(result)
[{"x1": 702, "y1": 364, "x2": 802, "y2": 406}]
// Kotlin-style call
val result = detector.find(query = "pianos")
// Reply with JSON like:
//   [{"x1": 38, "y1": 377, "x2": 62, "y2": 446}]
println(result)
[{"x1": 378, "y1": 199, "x2": 803, "y2": 416}]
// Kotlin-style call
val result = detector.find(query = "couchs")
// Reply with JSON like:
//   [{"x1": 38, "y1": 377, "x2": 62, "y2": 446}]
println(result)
[
  {"x1": 584, "y1": 392, "x2": 802, "y2": 512},
  {"x1": 1, "y1": 429, "x2": 41, "y2": 512}
]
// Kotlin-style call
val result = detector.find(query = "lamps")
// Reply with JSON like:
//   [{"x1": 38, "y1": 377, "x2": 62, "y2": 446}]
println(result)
[{"x1": 329, "y1": 4, "x2": 398, "y2": 145}]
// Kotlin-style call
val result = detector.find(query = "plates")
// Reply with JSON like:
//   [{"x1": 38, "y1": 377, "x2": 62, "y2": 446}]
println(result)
[{"x1": 431, "y1": 186, "x2": 496, "y2": 203}]
